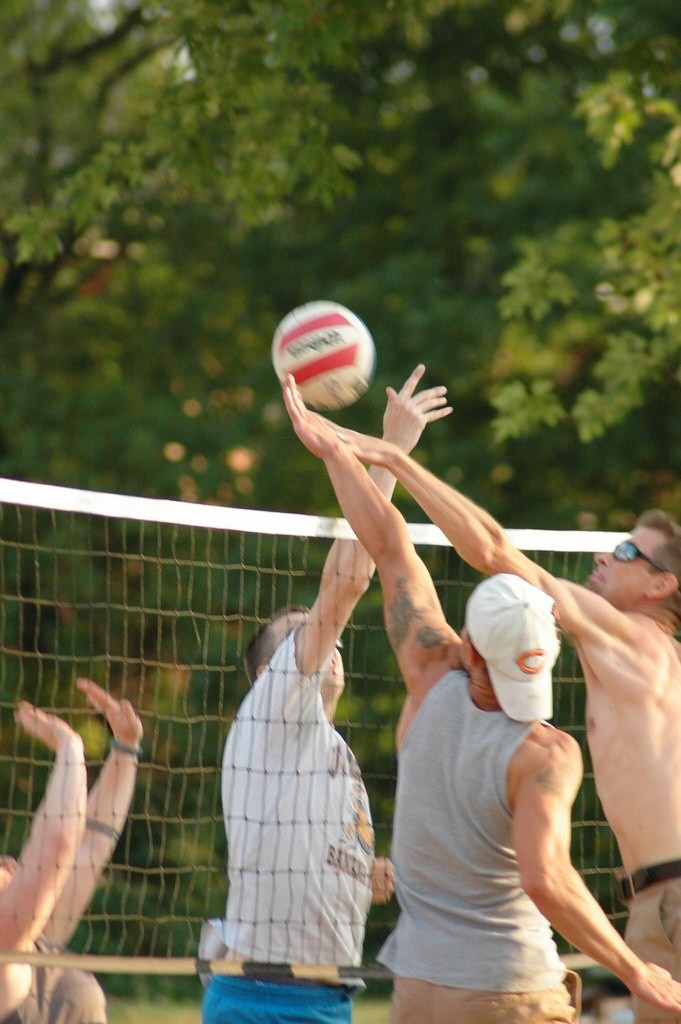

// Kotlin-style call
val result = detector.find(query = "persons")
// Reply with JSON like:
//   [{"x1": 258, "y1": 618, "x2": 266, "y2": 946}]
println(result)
[
  {"x1": 282, "y1": 373, "x2": 679, "y2": 1024},
  {"x1": 302, "y1": 408, "x2": 680, "y2": 1024},
  {"x1": 0, "y1": 675, "x2": 144, "y2": 1024},
  {"x1": 200, "y1": 363, "x2": 455, "y2": 1019}
]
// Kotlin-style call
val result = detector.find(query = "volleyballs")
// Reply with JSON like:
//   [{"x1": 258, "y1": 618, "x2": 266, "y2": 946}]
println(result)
[{"x1": 270, "y1": 299, "x2": 378, "y2": 412}]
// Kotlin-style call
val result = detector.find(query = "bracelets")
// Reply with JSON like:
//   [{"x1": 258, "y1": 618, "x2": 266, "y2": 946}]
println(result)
[{"x1": 109, "y1": 735, "x2": 143, "y2": 756}]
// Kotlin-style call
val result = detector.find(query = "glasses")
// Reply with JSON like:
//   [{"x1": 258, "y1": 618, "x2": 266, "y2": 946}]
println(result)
[
  {"x1": 614, "y1": 541, "x2": 681, "y2": 592},
  {"x1": 335, "y1": 639, "x2": 344, "y2": 648}
]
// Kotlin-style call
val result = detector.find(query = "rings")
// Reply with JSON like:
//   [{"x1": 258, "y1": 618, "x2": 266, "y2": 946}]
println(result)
[{"x1": 337, "y1": 431, "x2": 346, "y2": 439}]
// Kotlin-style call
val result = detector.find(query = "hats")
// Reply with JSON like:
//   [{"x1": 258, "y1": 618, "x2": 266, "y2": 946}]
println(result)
[{"x1": 465, "y1": 572, "x2": 561, "y2": 722}]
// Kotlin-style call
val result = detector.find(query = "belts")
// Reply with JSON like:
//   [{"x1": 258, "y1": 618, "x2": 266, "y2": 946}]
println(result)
[{"x1": 615, "y1": 860, "x2": 681, "y2": 901}]
